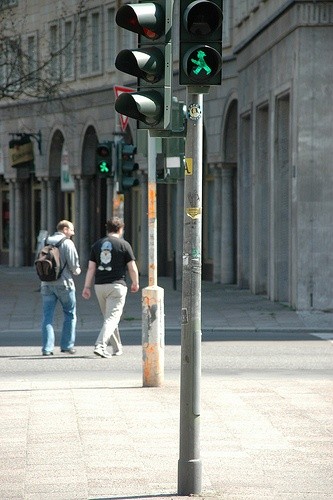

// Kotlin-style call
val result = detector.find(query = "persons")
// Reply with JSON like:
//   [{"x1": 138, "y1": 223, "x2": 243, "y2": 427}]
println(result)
[
  {"x1": 81, "y1": 216, "x2": 140, "y2": 359},
  {"x1": 33, "y1": 219, "x2": 81, "y2": 356}
]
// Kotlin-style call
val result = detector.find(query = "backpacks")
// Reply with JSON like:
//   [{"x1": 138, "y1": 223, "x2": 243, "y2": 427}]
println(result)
[{"x1": 34, "y1": 236, "x2": 70, "y2": 282}]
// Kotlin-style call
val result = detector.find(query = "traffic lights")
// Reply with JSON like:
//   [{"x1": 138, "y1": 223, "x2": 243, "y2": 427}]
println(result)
[
  {"x1": 116, "y1": 142, "x2": 139, "y2": 194},
  {"x1": 115, "y1": 0, "x2": 173, "y2": 131},
  {"x1": 96, "y1": 142, "x2": 112, "y2": 177},
  {"x1": 179, "y1": 0, "x2": 223, "y2": 92}
]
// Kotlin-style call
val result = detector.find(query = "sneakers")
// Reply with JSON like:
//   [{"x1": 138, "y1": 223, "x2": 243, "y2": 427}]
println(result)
[
  {"x1": 111, "y1": 351, "x2": 123, "y2": 356},
  {"x1": 94, "y1": 348, "x2": 112, "y2": 358}
]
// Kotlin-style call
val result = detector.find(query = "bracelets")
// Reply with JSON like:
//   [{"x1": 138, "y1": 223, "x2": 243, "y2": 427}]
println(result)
[{"x1": 83, "y1": 286, "x2": 92, "y2": 289}]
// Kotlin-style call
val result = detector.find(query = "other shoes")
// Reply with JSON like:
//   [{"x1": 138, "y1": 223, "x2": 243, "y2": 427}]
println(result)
[
  {"x1": 60, "y1": 348, "x2": 77, "y2": 354},
  {"x1": 42, "y1": 352, "x2": 54, "y2": 356}
]
